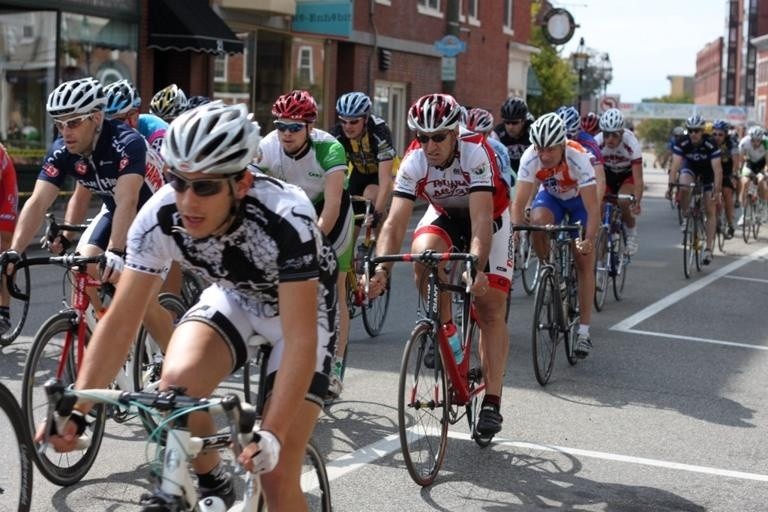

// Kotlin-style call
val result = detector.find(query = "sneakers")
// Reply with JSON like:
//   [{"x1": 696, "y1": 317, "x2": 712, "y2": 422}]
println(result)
[
  {"x1": 622, "y1": 232, "x2": 640, "y2": 256},
  {"x1": 700, "y1": 249, "x2": 713, "y2": 266},
  {"x1": 327, "y1": 370, "x2": 343, "y2": 399},
  {"x1": 476, "y1": 401, "x2": 505, "y2": 435},
  {"x1": 190, "y1": 463, "x2": 238, "y2": 512},
  {"x1": 665, "y1": 191, "x2": 768, "y2": 240},
  {"x1": 574, "y1": 332, "x2": 593, "y2": 356},
  {"x1": 424, "y1": 342, "x2": 443, "y2": 369},
  {"x1": 0, "y1": 316, "x2": 13, "y2": 335}
]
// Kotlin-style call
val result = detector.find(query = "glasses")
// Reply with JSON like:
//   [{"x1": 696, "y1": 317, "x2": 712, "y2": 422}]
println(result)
[
  {"x1": 337, "y1": 116, "x2": 364, "y2": 126},
  {"x1": 602, "y1": 130, "x2": 621, "y2": 139},
  {"x1": 163, "y1": 168, "x2": 244, "y2": 197},
  {"x1": 567, "y1": 132, "x2": 578, "y2": 139},
  {"x1": 413, "y1": 129, "x2": 452, "y2": 144},
  {"x1": 503, "y1": 118, "x2": 523, "y2": 126},
  {"x1": 52, "y1": 112, "x2": 97, "y2": 129},
  {"x1": 272, "y1": 119, "x2": 310, "y2": 134}
]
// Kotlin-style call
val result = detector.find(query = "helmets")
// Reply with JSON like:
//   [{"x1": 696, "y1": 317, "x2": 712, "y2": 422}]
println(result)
[
  {"x1": 334, "y1": 90, "x2": 373, "y2": 120},
  {"x1": 580, "y1": 111, "x2": 599, "y2": 133},
  {"x1": 147, "y1": 83, "x2": 188, "y2": 122},
  {"x1": 159, "y1": 98, "x2": 265, "y2": 176},
  {"x1": 598, "y1": 107, "x2": 626, "y2": 134},
  {"x1": 554, "y1": 104, "x2": 582, "y2": 133},
  {"x1": 528, "y1": 112, "x2": 568, "y2": 148},
  {"x1": 670, "y1": 114, "x2": 765, "y2": 141},
  {"x1": 464, "y1": 108, "x2": 495, "y2": 133},
  {"x1": 500, "y1": 96, "x2": 527, "y2": 120},
  {"x1": 405, "y1": 93, "x2": 463, "y2": 133},
  {"x1": 45, "y1": 75, "x2": 109, "y2": 120},
  {"x1": 269, "y1": 90, "x2": 320, "y2": 124},
  {"x1": 187, "y1": 95, "x2": 211, "y2": 111},
  {"x1": 102, "y1": 77, "x2": 142, "y2": 123}
]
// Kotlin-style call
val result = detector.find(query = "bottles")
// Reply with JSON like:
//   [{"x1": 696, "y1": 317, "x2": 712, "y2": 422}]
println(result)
[
  {"x1": 354, "y1": 243, "x2": 368, "y2": 275},
  {"x1": 439, "y1": 322, "x2": 465, "y2": 366},
  {"x1": 195, "y1": 495, "x2": 228, "y2": 512}
]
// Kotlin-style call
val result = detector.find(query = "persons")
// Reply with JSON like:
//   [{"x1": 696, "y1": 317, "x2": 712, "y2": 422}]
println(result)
[
  {"x1": 30, "y1": 98, "x2": 337, "y2": 511},
  {"x1": 0, "y1": 77, "x2": 183, "y2": 447},
  {"x1": 0, "y1": 140, "x2": 20, "y2": 336},
  {"x1": 648, "y1": 113, "x2": 768, "y2": 266}
]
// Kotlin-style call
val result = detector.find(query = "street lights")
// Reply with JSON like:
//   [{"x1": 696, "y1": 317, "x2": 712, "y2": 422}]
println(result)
[
  {"x1": 599, "y1": 52, "x2": 613, "y2": 99},
  {"x1": 571, "y1": 38, "x2": 588, "y2": 116},
  {"x1": 79, "y1": 16, "x2": 96, "y2": 80}
]
